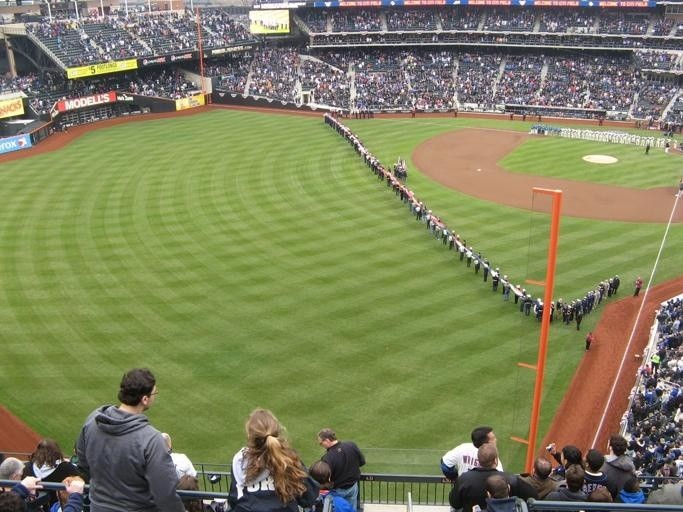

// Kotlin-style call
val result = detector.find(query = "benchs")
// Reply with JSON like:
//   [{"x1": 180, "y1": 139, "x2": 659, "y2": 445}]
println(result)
[{"x1": 0, "y1": 1, "x2": 683, "y2": 134}]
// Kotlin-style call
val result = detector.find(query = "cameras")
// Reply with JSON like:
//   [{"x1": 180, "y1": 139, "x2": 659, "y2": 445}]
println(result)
[{"x1": 546, "y1": 444, "x2": 552, "y2": 451}]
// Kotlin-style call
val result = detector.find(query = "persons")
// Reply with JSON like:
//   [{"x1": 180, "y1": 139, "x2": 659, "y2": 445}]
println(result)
[
  {"x1": 550, "y1": 275, "x2": 621, "y2": 330},
  {"x1": 227, "y1": 405, "x2": 321, "y2": 512},
  {"x1": 631, "y1": 276, "x2": 644, "y2": 298},
  {"x1": 74, "y1": 367, "x2": 186, "y2": 512},
  {"x1": 366, "y1": 155, "x2": 544, "y2": 322},
  {"x1": 315, "y1": 428, "x2": 367, "y2": 511},
  {"x1": 0, "y1": 432, "x2": 199, "y2": 512},
  {"x1": 0, "y1": 0, "x2": 681, "y2": 156},
  {"x1": 438, "y1": 298, "x2": 682, "y2": 512},
  {"x1": 297, "y1": 461, "x2": 355, "y2": 511}
]
